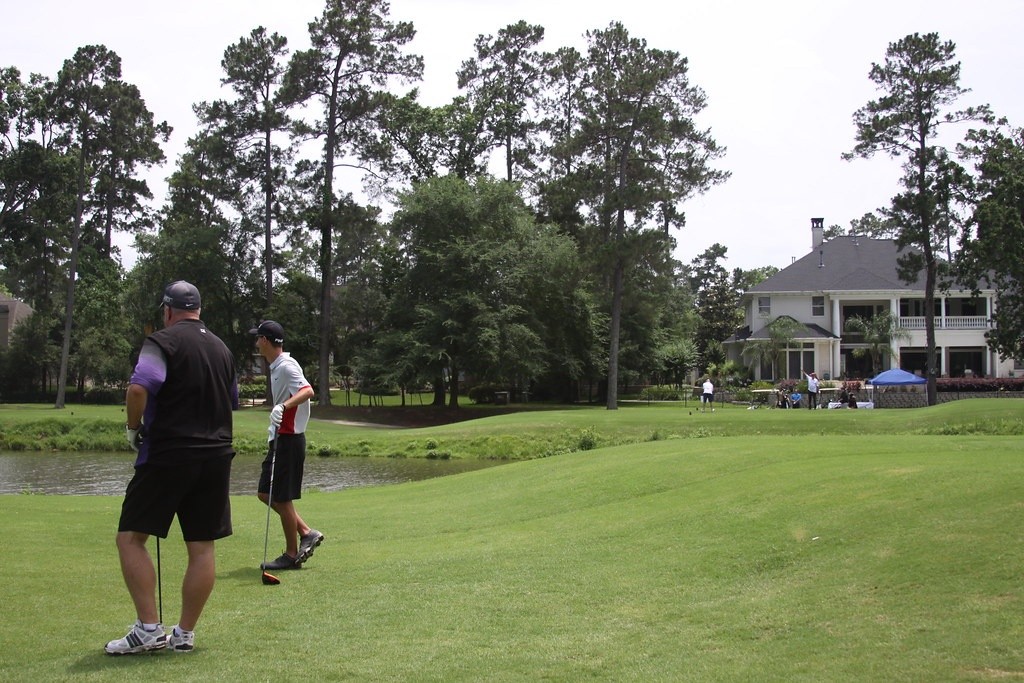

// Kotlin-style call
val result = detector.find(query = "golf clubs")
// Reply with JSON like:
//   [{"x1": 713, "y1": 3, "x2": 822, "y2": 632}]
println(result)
[
  {"x1": 261, "y1": 423, "x2": 280, "y2": 585},
  {"x1": 156, "y1": 536, "x2": 166, "y2": 630}
]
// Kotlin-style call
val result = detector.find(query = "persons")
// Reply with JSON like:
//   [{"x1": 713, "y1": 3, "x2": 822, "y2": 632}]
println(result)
[
  {"x1": 250, "y1": 319, "x2": 325, "y2": 571},
  {"x1": 910, "y1": 384, "x2": 916, "y2": 392},
  {"x1": 838, "y1": 388, "x2": 857, "y2": 409},
  {"x1": 791, "y1": 389, "x2": 802, "y2": 408},
  {"x1": 800, "y1": 367, "x2": 820, "y2": 410},
  {"x1": 884, "y1": 384, "x2": 896, "y2": 393},
  {"x1": 702, "y1": 378, "x2": 716, "y2": 412},
  {"x1": 900, "y1": 385, "x2": 907, "y2": 392},
  {"x1": 103, "y1": 280, "x2": 239, "y2": 654}
]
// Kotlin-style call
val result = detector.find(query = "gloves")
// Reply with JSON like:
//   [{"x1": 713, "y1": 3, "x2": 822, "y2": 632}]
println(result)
[
  {"x1": 270, "y1": 404, "x2": 284, "y2": 427},
  {"x1": 126, "y1": 422, "x2": 144, "y2": 452}
]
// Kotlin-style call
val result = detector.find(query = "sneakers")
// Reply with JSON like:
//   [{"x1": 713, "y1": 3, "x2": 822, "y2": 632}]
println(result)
[
  {"x1": 260, "y1": 549, "x2": 302, "y2": 569},
  {"x1": 104, "y1": 619, "x2": 168, "y2": 654},
  {"x1": 166, "y1": 625, "x2": 194, "y2": 652},
  {"x1": 295, "y1": 529, "x2": 324, "y2": 565}
]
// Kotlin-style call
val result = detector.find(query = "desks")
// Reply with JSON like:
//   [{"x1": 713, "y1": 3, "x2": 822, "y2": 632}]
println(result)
[
  {"x1": 819, "y1": 388, "x2": 841, "y2": 405},
  {"x1": 751, "y1": 390, "x2": 779, "y2": 410}
]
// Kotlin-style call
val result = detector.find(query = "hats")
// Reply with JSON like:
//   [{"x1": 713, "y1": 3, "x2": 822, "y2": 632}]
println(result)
[
  {"x1": 159, "y1": 280, "x2": 201, "y2": 309},
  {"x1": 249, "y1": 320, "x2": 284, "y2": 342}
]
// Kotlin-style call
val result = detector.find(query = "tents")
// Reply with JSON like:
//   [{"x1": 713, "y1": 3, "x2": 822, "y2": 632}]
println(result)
[{"x1": 868, "y1": 368, "x2": 929, "y2": 405}]
